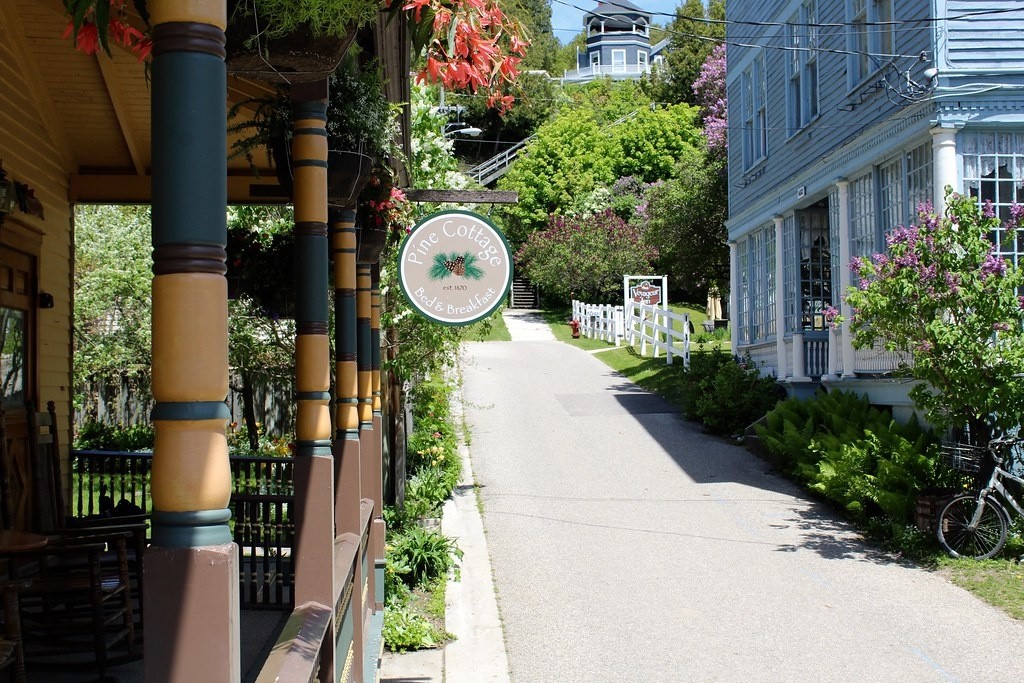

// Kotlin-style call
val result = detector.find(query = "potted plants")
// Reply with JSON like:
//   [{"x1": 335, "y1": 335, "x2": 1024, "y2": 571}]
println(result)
[{"x1": 368, "y1": 463, "x2": 463, "y2": 678}]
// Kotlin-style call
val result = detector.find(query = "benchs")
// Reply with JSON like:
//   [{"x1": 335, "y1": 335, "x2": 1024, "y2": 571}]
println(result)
[{"x1": 700, "y1": 320, "x2": 715, "y2": 332}]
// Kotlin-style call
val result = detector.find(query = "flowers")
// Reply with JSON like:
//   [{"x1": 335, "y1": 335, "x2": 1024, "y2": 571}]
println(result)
[
  {"x1": 200, "y1": 218, "x2": 309, "y2": 268},
  {"x1": 237, "y1": 43, "x2": 414, "y2": 168},
  {"x1": 364, "y1": 180, "x2": 417, "y2": 239}
]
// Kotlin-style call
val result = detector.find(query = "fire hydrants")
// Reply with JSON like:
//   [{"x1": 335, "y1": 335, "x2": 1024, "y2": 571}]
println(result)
[{"x1": 568, "y1": 317, "x2": 582, "y2": 338}]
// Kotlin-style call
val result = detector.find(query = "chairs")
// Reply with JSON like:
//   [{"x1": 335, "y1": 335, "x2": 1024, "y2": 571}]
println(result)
[{"x1": 0, "y1": 400, "x2": 152, "y2": 683}]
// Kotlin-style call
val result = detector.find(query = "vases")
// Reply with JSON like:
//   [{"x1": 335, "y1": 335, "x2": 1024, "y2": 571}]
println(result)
[
  {"x1": 132, "y1": 0, "x2": 385, "y2": 321},
  {"x1": 913, "y1": 486, "x2": 965, "y2": 537}
]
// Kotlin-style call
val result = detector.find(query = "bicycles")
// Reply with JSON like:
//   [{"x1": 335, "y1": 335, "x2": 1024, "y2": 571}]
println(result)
[{"x1": 932, "y1": 434, "x2": 1024, "y2": 559}]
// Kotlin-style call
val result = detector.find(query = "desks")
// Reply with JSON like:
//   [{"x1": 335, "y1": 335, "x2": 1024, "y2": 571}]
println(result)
[{"x1": 0, "y1": 530, "x2": 48, "y2": 683}]
